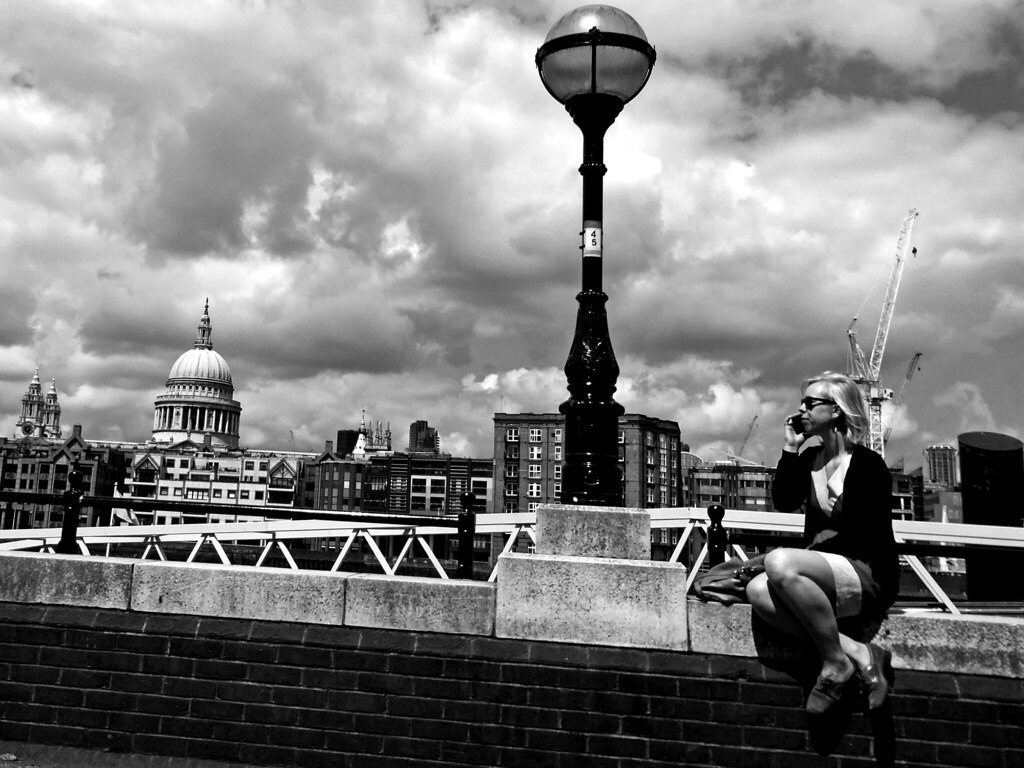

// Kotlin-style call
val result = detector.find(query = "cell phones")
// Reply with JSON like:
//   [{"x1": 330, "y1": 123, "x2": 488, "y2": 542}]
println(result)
[{"x1": 791, "y1": 415, "x2": 805, "y2": 434}]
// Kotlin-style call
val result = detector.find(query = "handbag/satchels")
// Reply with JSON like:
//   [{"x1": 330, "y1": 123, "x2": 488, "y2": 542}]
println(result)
[{"x1": 695, "y1": 558, "x2": 765, "y2": 606}]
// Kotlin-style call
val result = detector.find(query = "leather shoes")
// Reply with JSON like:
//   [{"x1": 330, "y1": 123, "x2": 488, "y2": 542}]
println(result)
[
  {"x1": 860, "y1": 643, "x2": 888, "y2": 710},
  {"x1": 806, "y1": 657, "x2": 855, "y2": 715}
]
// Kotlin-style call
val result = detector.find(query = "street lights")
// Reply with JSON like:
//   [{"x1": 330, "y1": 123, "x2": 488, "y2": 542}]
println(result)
[{"x1": 538, "y1": 4, "x2": 656, "y2": 504}]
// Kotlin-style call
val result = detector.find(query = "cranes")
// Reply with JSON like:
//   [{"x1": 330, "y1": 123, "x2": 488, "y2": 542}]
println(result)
[{"x1": 844, "y1": 207, "x2": 925, "y2": 461}]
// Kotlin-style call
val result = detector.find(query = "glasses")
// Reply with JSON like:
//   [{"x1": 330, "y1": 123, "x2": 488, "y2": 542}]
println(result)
[{"x1": 800, "y1": 396, "x2": 837, "y2": 409}]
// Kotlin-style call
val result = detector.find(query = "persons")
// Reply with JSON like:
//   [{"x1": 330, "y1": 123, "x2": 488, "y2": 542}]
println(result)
[{"x1": 746, "y1": 369, "x2": 901, "y2": 714}]
